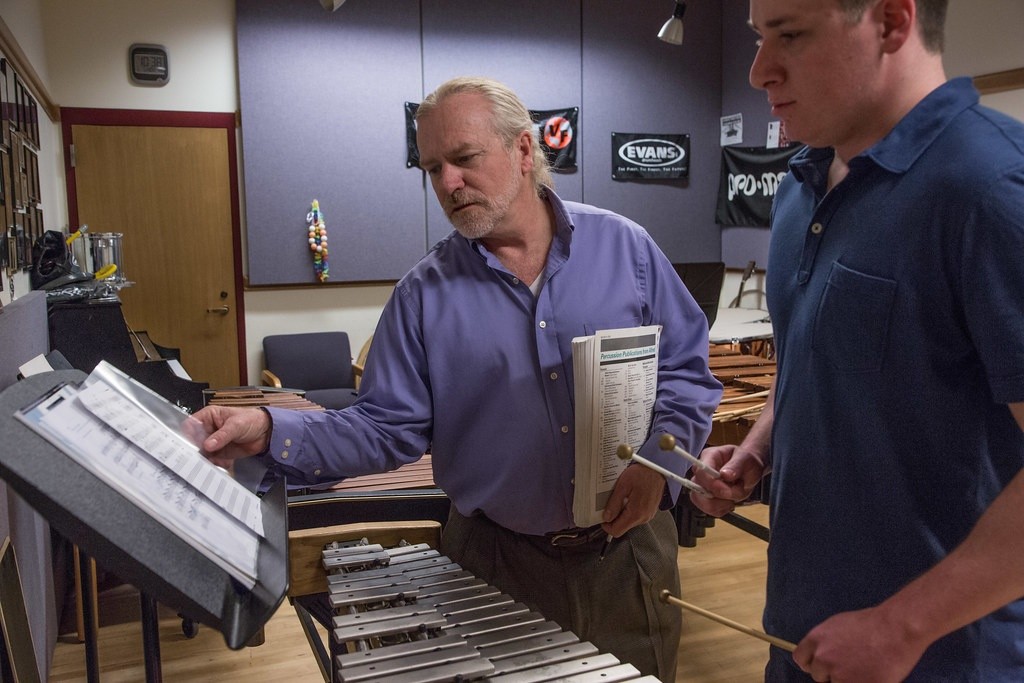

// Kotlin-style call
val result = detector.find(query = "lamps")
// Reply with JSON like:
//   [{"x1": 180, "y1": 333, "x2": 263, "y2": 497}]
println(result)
[{"x1": 657, "y1": 0, "x2": 687, "y2": 46}]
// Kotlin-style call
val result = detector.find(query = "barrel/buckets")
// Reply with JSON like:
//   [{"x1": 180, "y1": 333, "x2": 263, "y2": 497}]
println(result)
[{"x1": 90, "y1": 231, "x2": 124, "y2": 281}]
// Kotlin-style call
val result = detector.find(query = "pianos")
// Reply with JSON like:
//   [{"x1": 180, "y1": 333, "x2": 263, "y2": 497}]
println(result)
[{"x1": 44, "y1": 286, "x2": 212, "y2": 416}]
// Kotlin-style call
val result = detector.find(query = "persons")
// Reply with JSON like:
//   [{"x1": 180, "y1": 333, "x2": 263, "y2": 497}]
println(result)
[
  {"x1": 688, "y1": 0, "x2": 1024, "y2": 682},
  {"x1": 178, "y1": 77, "x2": 724, "y2": 683}
]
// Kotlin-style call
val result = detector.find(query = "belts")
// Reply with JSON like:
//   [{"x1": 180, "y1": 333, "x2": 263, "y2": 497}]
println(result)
[{"x1": 478, "y1": 512, "x2": 608, "y2": 547}]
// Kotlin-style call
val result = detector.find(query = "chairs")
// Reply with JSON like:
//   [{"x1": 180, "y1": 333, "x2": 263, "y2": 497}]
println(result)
[{"x1": 262, "y1": 332, "x2": 365, "y2": 410}]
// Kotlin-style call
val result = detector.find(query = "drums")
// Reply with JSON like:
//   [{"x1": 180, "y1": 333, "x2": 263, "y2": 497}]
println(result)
[{"x1": 68, "y1": 231, "x2": 125, "y2": 285}]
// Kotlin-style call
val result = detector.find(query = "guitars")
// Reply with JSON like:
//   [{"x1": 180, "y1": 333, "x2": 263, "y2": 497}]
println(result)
[{"x1": 736, "y1": 260, "x2": 756, "y2": 308}]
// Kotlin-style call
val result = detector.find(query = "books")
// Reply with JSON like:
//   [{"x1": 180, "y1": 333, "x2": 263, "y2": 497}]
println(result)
[
  {"x1": 13, "y1": 358, "x2": 268, "y2": 590},
  {"x1": 568, "y1": 324, "x2": 664, "y2": 526}
]
